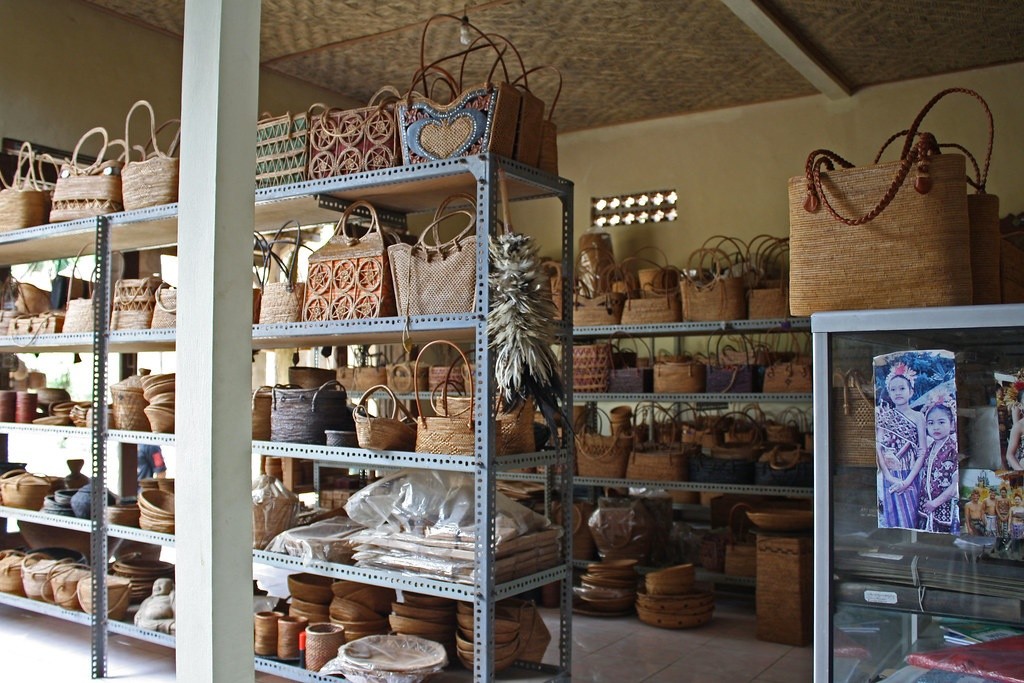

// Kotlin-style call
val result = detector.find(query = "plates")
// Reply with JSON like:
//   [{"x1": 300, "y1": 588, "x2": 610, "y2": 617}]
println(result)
[{"x1": 112, "y1": 559, "x2": 173, "y2": 601}]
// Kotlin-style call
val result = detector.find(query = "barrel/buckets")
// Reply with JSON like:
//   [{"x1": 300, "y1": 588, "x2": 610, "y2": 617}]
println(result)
[{"x1": 1, "y1": 470, "x2": 131, "y2": 620}]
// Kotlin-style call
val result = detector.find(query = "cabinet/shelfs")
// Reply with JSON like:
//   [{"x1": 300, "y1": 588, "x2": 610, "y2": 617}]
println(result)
[{"x1": 0, "y1": 150, "x2": 814, "y2": 683}]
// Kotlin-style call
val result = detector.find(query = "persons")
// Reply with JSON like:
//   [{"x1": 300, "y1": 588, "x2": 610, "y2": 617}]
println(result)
[
  {"x1": 136, "y1": 444, "x2": 168, "y2": 492},
  {"x1": 964, "y1": 486, "x2": 1024, "y2": 539},
  {"x1": 996, "y1": 383, "x2": 1024, "y2": 471},
  {"x1": 134, "y1": 578, "x2": 176, "y2": 635},
  {"x1": 877, "y1": 364, "x2": 960, "y2": 532}
]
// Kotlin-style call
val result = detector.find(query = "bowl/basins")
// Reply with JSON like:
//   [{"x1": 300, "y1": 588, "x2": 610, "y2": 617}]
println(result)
[
  {"x1": 577, "y1": 559, "x2": 636, "y2": 612},
  {"x1": 138, "y1": 489, "x2": 174, "y2": 534},
  {"x1": 141, "y1": 373, "x2": 175, "y2": 434},
  {"x1": 645, "y1": 564, "x2": 696, "y2": 593},
  {"x1": 285, "y1": 575, "x2": 531, "y2": 676}
]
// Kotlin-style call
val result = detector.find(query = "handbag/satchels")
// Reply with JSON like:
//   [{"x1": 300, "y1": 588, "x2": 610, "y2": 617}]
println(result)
[{"x1": 0, "y1": 14, "x2": 1024, "y2": 683}]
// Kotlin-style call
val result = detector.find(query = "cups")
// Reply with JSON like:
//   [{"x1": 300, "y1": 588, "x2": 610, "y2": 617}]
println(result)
[
  {"x1": 0, "y1": 391, "x2": 15, "y2": 422},
  {"x1": 252, "y1": 611, "x2": 347, "y2": 673},
  {"x1": 14, "y1": 392, "x2": 37, "y2": 425}
]
